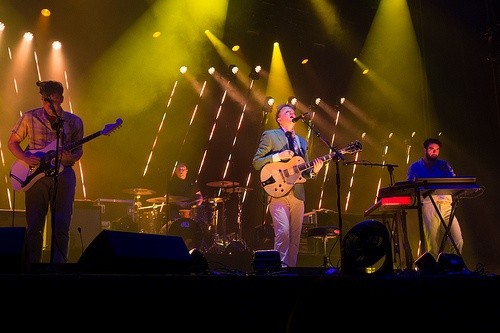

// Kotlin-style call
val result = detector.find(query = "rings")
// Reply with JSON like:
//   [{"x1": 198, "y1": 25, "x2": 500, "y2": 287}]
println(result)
[{"x1": 289, "y1": 151, "x2": 291, "y2": 154}]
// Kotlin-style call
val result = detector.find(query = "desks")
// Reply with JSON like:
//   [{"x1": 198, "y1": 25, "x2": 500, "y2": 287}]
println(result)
[{"x1": 413, "y1": 183, "x2": 488, "y2": 260}]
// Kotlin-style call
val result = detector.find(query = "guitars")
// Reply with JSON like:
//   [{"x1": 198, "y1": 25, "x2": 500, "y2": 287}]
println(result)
[
  {"x1": 259, "y1": 140, "x2": 362, "y2": 198},
  {"x1": 10, "y1": 117, "x2": 125, "y2": 192}
]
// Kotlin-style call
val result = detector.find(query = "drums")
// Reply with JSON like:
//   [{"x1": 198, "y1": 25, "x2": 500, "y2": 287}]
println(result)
[
  {"x1": 179, "y1": 209, "x2": 194, "y2": 221},
  {"x1": 138, "y1": 204, "x2": 167, "y2": 234},
  {"x1": 161, "y1": 218, "x2": 204, "y2": 252}
]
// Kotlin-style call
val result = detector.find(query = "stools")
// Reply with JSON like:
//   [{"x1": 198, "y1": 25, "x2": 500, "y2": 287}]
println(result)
[{"x1": 307, "y1": 227, "x2": 340, "y2": 267}]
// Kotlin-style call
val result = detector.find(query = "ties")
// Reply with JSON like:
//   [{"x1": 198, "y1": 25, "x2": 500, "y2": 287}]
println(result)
[{"x1": 285, "y1": 131, "x2": 295, "y2": 157}]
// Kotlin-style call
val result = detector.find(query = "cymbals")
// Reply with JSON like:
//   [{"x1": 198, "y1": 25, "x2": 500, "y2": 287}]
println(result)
[
  {"x1": 146, "y1": 196, "x2": 191, "y2": 203},
  {"x1": 223, "y1": 188, "x2": 255, "y2": 193},
  {"x1": 122, "y1": 188, "x2": 155, "y2": 195},
  {"x1": 207, "y1": 181, "x2": 239, "y2": 187}
]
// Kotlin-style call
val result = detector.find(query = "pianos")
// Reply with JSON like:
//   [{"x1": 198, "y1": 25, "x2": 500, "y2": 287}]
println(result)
[{"x1": 363, "y1": 176, "x2": 481, "y2": 270}]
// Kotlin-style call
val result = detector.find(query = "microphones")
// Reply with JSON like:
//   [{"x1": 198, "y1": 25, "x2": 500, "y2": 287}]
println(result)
[
  {"x1": 343, "y1": 162, "x2": 352, "y2": 165},
  {"x1": 292, "y1": 112, "x2": 308, "y2": 123},
  {"x1": 44, "y1": 98, "x2": 54, "y2": 102},
  {"x1": 78, "y1": 228, "x2": 84, "y2": 253}
]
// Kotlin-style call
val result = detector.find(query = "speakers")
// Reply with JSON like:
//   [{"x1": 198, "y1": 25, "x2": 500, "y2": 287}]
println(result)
[
  {"x1": 77, "y1": 229, "x2": 191, "y2": 269},
  {"x1": 0, "y1": 227, "x2": 27, "y2": 264},
  {"x1": 45, "y1": 205, "x2": 101, "y2": 263}
]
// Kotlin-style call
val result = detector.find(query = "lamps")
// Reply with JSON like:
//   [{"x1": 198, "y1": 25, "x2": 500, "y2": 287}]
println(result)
[
  {"x1": 253, "y1": 250, "x2": 281, "y2": 273},
  {"x1": 415, "y1": 252, "x2": 436, "y2": 271},
  {"x1": 199, "y1": 39, "x2": 443, "y2": 140}
]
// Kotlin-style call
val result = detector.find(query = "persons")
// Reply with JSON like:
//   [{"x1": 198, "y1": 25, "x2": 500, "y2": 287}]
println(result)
[
  {"x1": 253, "y1": 104, "x2": 324, "y2": 268},
  {"x1": 8, "y1": 80, "x2": 83, "y2": 264},
  {"x1": 167, "y1": 164, "x2": 203, "y2": 210},
  {"x1": 407, "y1": 138, "x2": 464, "y2": 260}
]
